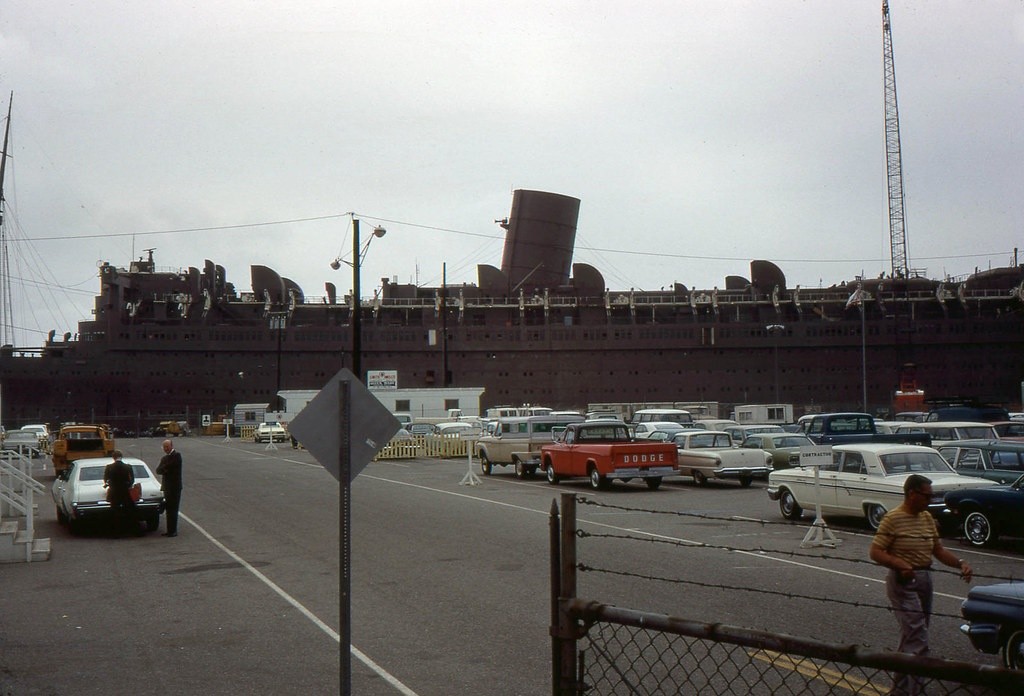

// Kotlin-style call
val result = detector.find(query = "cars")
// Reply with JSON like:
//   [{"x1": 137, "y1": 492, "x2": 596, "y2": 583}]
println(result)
[
  {"x1": 475, "y1": 415, "x2": 587, "y2": 480},
  {"x1": 944, "y1": 471, "x2": 1024, "y2": 550},
  {"x1": 765, "y1": 443, "x2": 1001, "y2": 531},
  {"x1": 374, "y1": 399, "x2": 1024, "y2": 487},
  {"x1": 960, "y1": 579, "x2": 1024, "y2": 674},
  {"x1": 0, "y1": 419, "x2": 116, "y2": 480},
  {"x1": 255, "y1": 422, "x2": 286, "y2": 444},
  {"x1": 51, "y1": 457, "x2": 166, "y2": 535}
]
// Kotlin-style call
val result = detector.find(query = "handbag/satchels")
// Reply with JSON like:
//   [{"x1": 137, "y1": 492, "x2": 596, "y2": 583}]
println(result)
[{"x1": 128, "y1": 484, "x2": 142, "y2": 504}]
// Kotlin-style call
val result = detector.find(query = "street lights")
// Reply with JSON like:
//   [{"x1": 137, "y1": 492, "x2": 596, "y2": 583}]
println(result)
[
  {"x1": 329, "y1": 219, "x2": 386, "y2": 379},
  {"x1": 765, "y1": 324, "x2": 786, "y2": 403},
  {"x1": 268, "y1": 304, "x2": 289, "y2": 413}
]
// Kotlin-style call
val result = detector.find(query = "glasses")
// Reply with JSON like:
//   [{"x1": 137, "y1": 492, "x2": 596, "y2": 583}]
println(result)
[{"x1": 915, "y1": 492, "x2": 935, "y2": 499}]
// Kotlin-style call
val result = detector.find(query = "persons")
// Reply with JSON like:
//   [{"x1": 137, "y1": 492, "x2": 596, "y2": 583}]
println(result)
[
  {"x1": 104, "y1": 450, "x2": 134, "y2": 539},
  {"x1": 156, "y1": 439, "x2": 182, "y2": 538},
  {"x1": 870, "y1": 475, "x2": 972, "y2": 696}
]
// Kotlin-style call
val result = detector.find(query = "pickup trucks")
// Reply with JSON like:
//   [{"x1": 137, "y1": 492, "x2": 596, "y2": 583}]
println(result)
[{"x1": 539, "y1": 422, "x2": 681, "y2": 492}]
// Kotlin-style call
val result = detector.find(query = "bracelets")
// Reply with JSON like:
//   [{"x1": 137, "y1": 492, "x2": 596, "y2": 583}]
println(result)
[{"x1": 958, "y1": 558, "x2": 963, "y2": 562}]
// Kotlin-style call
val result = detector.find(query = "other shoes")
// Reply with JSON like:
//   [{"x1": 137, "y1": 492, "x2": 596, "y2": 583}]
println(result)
[{"x1": 162, "y1": 532, "x2": 177, "y2": 537}]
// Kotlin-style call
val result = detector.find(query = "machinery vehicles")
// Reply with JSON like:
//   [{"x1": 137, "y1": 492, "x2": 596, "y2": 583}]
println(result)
[{"x1": 876, "y1": 1, "x2": 929, "y2": 414}]
